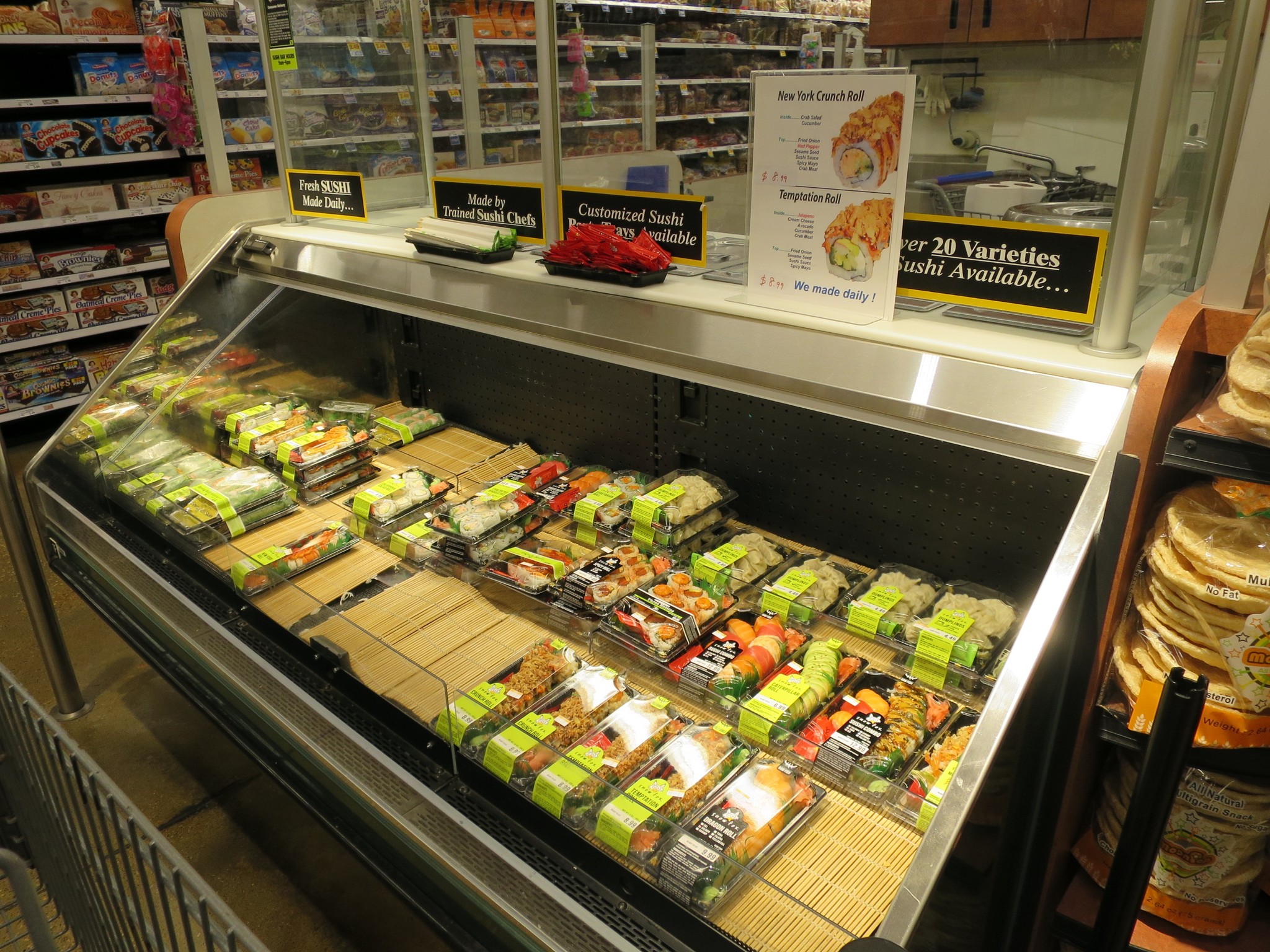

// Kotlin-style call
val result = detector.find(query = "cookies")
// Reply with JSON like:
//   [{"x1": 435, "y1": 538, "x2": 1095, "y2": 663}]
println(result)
[
  {"x1": 0, "y1": 295, "x2": 68, "y2": 338},
  {"x1": 41, "y1": 246, "x2": 153, "y2": 279},
  {"x1": 0, "y1": 242, "x2": 30, "y2": 284},
  {"x1": 81, "y1": 280, "x2": 149, "y2": 322}
]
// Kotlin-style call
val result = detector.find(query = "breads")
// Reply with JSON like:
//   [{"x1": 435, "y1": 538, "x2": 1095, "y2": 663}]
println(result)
[{"x1": 748, "y1": 0, "x2": 871, "y2": 18}]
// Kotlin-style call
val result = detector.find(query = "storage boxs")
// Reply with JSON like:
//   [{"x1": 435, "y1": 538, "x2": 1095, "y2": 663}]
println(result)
[{"x1": 61, "y1": 389, "x2": 1024, "y2": 908}]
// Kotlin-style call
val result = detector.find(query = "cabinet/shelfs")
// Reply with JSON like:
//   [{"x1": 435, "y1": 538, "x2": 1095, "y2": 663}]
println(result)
[
  {"x1": 1028, "y1": 286, "x2": 1270, "y2": 952},
  {"x1": 0, "y1": 0, "x2": 869, "y2": 424},
  {"x1": 867, "y1": 0, "x2": 1089, "y2": 47},
  {"x1": 1085, "y1": 0, "x2": 1148, "y2": 40}
]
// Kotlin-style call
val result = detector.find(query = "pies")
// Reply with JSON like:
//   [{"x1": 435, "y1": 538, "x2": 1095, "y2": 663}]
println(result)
[{"x1": 1094, "y1": 312, "x2": 1270, "y2": 908}]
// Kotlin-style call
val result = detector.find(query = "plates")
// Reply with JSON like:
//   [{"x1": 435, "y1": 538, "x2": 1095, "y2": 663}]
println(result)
[
  {"x1": 404, "y1": 240, "x2": 523, "y2": 263},
  {"x1": 535, "y1": 258, "x2": 677, "y2": 287}
]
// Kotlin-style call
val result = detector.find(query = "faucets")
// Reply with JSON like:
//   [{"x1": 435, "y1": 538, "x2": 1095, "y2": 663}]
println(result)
[{"x1": 971, "y1": 143, "x2": 1058, "y2": 180}]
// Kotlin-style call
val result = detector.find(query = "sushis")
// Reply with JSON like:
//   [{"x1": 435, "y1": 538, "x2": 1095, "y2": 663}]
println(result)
[
  {"x1": 821, "y1": 198, "x2": 894, "y2": 283},
  {"x1": 831, "y1": 90, "x2": 904, "y2": 189},
  {"x1": 121, "y1": 392, "x2": 1019, "y2": 914}
]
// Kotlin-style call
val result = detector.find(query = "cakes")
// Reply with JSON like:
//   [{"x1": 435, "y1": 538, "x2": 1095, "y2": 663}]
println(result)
[
  {"x1": 16, "y1": 114, "x2": 176, "y2": 160},
  {"x1": 0, "y1": 186, "x2": 194, "y2": 224},
  {"x1": 204, "y1": 159, "x2": 257, "y2": 194},
  {"x1": 0, "y1": 0, "x2": 140, "y2": 35}
]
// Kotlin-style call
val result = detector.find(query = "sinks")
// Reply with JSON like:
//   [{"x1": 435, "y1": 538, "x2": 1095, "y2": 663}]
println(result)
[{"x1": 905, "y1": 182, "x2": 968, "y2": 215}]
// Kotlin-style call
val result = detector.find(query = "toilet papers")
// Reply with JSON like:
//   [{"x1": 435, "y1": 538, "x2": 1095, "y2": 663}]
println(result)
[
  {"x1": 960, "y1": 184, "x2": 1017, "y2": 220},
  {"x1": 1000, "y1": 179, "x2": 1047, "y2": 208}
]
